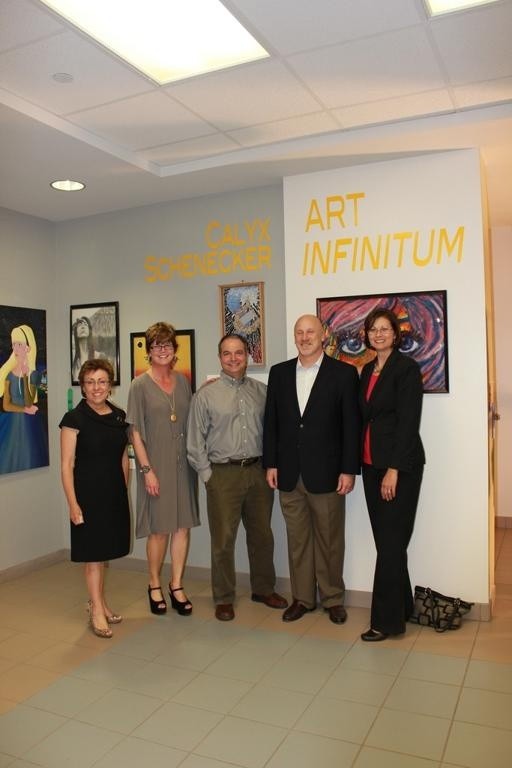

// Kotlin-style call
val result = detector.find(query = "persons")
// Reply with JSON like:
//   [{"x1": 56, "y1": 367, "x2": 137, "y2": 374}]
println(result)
[
  {"x1": 262, "y1": 315, "x2": 371, "y2": 626},
  {"x1": 126, "y1": 323, "x2": 204, "y2": 621},
  {"x1": 71, "y1": 316, "x2": 109, "y2": 379},
  {"x1": 357, "y1": 305, "x2": 429, "y2": 642},
  {"x1": 58, "y1": 356, "x2": 135, "y2": 639},
  {"x1": 186, "y1": 335, "x2": 287, "y2": 622}
]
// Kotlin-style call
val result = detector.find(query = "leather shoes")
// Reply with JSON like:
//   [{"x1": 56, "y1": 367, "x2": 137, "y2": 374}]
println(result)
[
  {"x1": 252, "y1": 592, "x2": 287, "y2": 609},
  {"x1": 215, "y1": 604, "x2": 234, "y2": 619},
  {"x1": 362, "y1": 628, "x2": 390, "y2": 641},
  {"x1": 282, "y1": 600, "x2": 315, "y2": 621},
  {"x1": 325, "y1": 604, "x2": 346, "y2": 624}
]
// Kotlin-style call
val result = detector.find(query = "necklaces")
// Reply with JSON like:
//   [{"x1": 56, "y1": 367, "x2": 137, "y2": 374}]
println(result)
[
  {"x1": 374, "y1": 361, "x2": 384, "y2": 374},
  {"x1": 151, "y1": 371, "x2": 177, "y2": 423}
]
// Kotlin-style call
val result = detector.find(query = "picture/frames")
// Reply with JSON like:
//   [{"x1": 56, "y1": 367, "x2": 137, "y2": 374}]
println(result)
[
  {"x1": 68, "y1": 301, "x2": 120, "y2": 386},
  {"x1": 218, "y1": 279, "x2": 266, "y2": 369},
  {"x1": 129, "y1": 327, "x2": 197, "y2": 397},
  {"x1": 314, "y1": 292, "x2": 452, "y2": 394}
]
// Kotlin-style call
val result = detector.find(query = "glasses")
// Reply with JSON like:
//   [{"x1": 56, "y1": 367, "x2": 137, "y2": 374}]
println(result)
[
  {"x1": 150, "y1": 344, "x2": 177, "y2": 353},
  {"x1": 366, "y1": 326, "x2": 394, "y2": 334}
]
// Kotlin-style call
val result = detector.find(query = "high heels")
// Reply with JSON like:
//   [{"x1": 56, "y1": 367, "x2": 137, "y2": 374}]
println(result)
[
  {"x1": 169, "y1": 584, "x2": 192, "y2": 615},
  {"x1": 90, "y1": 608, "x2": 112, "y2": 637},
  {"x1": 86, "y1": 600, "x2": 122, "y2": 624},
  {"x1": 148, "y1": 585, "x2": 166, "y2": 615}
]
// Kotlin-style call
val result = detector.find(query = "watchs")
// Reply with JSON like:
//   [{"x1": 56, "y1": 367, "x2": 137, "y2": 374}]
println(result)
[{"x1": 139, "y1": 464, "x2": 154, "y2": 474}]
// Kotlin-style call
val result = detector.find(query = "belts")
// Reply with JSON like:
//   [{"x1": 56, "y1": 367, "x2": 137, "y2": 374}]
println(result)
[{"x1": 214, "y1": 457, "x2": 263, "y2": 467}]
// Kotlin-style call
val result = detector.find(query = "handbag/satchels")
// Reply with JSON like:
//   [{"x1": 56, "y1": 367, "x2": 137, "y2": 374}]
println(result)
[{"x1": 408, "y1": 587, "x2": 471, "y2": 632}]
